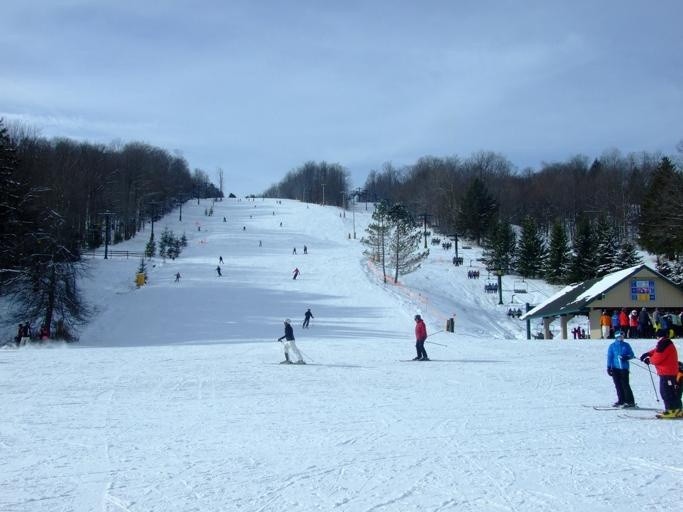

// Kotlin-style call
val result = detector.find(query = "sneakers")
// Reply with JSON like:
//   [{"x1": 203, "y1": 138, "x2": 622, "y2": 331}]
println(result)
[
  {"x1": 614, "y1": 399, "x2": 636, "y2": 408},
  {"x1": 657, "y1": 409, "x2": 682, "y2": 418}
]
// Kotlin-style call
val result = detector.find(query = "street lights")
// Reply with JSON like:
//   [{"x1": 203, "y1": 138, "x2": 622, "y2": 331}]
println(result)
[{"x1": 320, "y1": 182, "x2": 358, "y2": 242}]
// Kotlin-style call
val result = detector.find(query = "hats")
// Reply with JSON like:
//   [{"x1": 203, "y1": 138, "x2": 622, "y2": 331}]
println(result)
[
  {"x1": 615, "y1": 331, "x2": 625, "y2": 337},
  {"x1": 655, "y1": 328, "x2": 668, "y2": 338},
  {"x1": 283, "y1": 319, "x2": 291, "y2": 324}
]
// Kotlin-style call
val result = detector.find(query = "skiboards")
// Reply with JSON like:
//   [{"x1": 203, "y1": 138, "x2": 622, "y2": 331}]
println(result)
[
  {"x1": 582, "y1": 405, "x2": 620, "y2": 410},
  {"x1": 617, "y1": 413, "x2": 656, "y2": 420}
]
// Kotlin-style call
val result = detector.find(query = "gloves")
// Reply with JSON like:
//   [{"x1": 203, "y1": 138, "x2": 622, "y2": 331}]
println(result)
[
  {"x1": 639, "y1": 352, "x2": 650, "y2": 365},
  {"x1": 605, "y1": 366, "x2": 613, "y2": 377}
]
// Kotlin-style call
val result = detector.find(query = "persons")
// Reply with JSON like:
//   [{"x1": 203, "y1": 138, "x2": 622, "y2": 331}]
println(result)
[
  {"x1": 303, "y1": 309, "x2": 314, "y2": 328},
  {"x1": 278, "y1": 319, "x2": 304, "y2": 364},
  {"x1": 17, "y1": 319, "x2": 49, "y2": 347},
  {"x1": 174, "y1": 198, "x2": 314, "y2": 282},
  {"x1": 600, "y1": 307, "x2": 683, "y2": 339},
  {"x1": 606, "y1": 331, "x2": 635, "y2": 407},
  {"x1": 413, "y1": 314, "x2": 428, "y2": 361},
  {"x1": 423, "y1": 231, "x2": 586, "y2": 340},
  {"x1": 640, "y1": 329, "x2": 683, "y2": 418}
]
